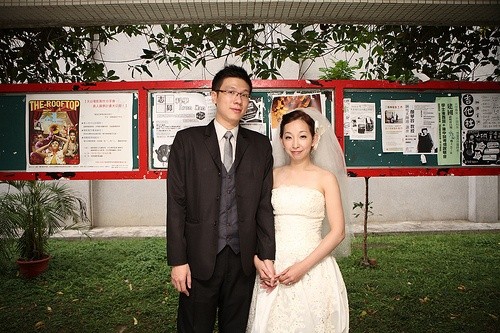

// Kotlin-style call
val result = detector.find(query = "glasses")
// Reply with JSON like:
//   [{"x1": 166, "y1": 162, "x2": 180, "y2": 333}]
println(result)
[{"x1": 215, "y1": 90, "x2": 252, "y2": 100}]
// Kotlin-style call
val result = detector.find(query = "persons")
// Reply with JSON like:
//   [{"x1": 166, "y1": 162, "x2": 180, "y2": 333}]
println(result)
[
  {"x1": 418, "y1": 128, "x2": 434, "y2": 153},
  {"x1": 166, "y1": 63, "x2": 279, "y2": 333},
  {"x1": 245, "y1": 107, "x2": 355, "y2": 333},
  {"x1": 32, "y1": 130, "x2": 78, "y2": 165}
]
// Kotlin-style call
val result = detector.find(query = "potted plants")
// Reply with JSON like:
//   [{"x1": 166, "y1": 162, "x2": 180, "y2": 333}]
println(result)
[{"x1": 0, "y1": 175, "x2": 92, "y2": 279}]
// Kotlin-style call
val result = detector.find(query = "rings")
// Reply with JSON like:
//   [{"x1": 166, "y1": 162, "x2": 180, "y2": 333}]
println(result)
[{"x1": 288, "y1": 282, "x2": 291, "y2": 285}]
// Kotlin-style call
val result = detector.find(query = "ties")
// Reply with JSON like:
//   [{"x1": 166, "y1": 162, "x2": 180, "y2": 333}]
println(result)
[{"x1": 223, "y1": 131, "x2": 233, "y2": 172}]
